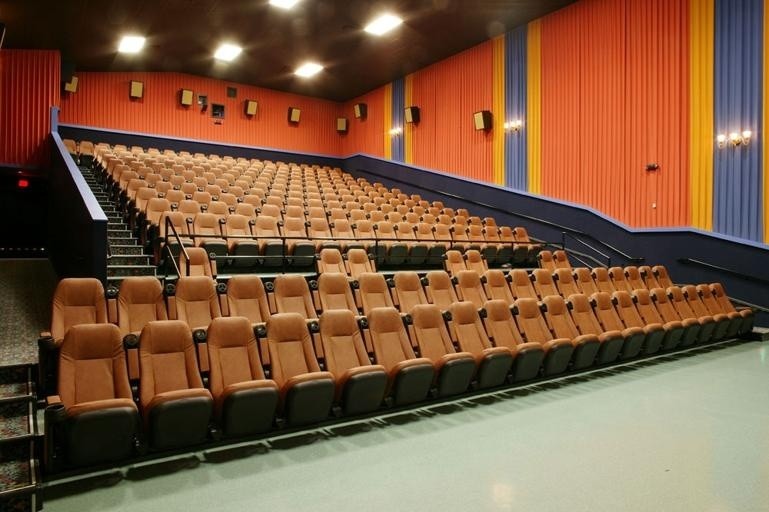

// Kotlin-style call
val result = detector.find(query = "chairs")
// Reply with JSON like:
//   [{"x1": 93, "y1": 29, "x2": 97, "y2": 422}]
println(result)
[
  {"x1": 277, "y1": 217, "x2": 309, "y2": 245},
  {"x1": 220, "y1": 215, "x2": 255, "y2": 253},
  {"x1": 450, "y1": 225, "x2": 469, "y2": 249},
  {"x1": 573, "y1": 267, "x2": 599, "y2": 297},
  {"x1": 125, "y1": 179, "x2": 148, "y2": 216},
  {"x1": 624, "y1": 264, "x2": 649, "y2": 291},
  {"x1": 652, "y1": 264, "x2": 675, "y2": 286},
  {"x1": 551, "y1": 267, "x2": 582, "y2": 300},
  {"x1": 266, "y1": 195, "x2": 283, "y2": 205},
  {"x1": 397, "y1": 205, "x2": 410, "y2": 215},
  {"x1": 466, "y1": 250, "x2": 488, "y2": 270},
  {"x1": 540, "y1": 295, "x2": 601, "y2": 371},
  {"x1": 632, "y1": 286, "x2": 685, "y2": 351},
  {"x1": 94, "y1": 143, "x2": 125, "y2": 153},
  {"x1": 364, "y1": 203, "x2": 376, "y2": 216},
  {"x1": 456, "y1": 209, "x2": 470, "y2": 222},
  {"x1": 482, "y1": 226, "x2": 500, "y2": 261},
  {"x1": 104, "y1": 157, "x2": 125, "y2": 192},
  {"x1": 305, "y1": 217, "x2": 334, "y2": 243},
  {"x1": 186, "y1": 214, "x2": 226, "y2": 248},
  {"x1": 529, "y1": 269, "x2": 560, "y2": 300},
  {"x1": 80, "y1": 142, "x2": 93, "y2": 157},
  {"x1": 373, "y1": 222, "x2": 409, "y2": 266},
  {"x1": 118, "y1": 275, "x2": 168, "y2": 336},
  {"x1": 242, "y1": 194, "x2": 261, "y2": 206},
  {"x1": 411, "y1": 194, "x2": 420, "y2": 203},
  {"x1": 117, "y1": 170, "x2": 139, "y2": 205},
  {"x1": 351, "y1": 208, "x2": 368, "y2": 221},
  {"x1": 406, "y1": 211, "x2": 419, "y2": 221},
  {"x1": 159, "y1": 211, "x2": 193, "y2": 248},
  {"x1": 285, "y1": 205, "x2": 305, "y2": 215},
  {"x1": 167, "y1": 188, "x2": 186, "y2": 204},
  {"x1": 609, "y1": 267, "x2": 631, "y2": 294},
  {"x1": 394, "y1": 271, "x2": 429, "y2": 313},
  {"x1": 589, "y1": 291, "x2": 646, "y2": 359},
  {"x1": 252, "y1": 216, "x2": 276, "y2": 252},
  {"x1": 206, "y1": 200, "x2": 230, "y2": 216},
  {"x1": 434, "y1": 201, "x2": 445, "y2": 209},
  {"x1": 453, "y1": 216, "x2": 467, "y2": 224},
  {"x1": 346, "y1": 248, "x2": 376, "y2": 278},
  {"x1": 484, "y1": 299, "x2": 545, "y2": 381},
  {"x1": 442, "y1": 207, "x2": 455, "y2": 214},
  {"x1": 218, "y1": 193, "x2": 237, "y2": 203},
  {"x1": 316, "y1": 274, "x2": 359, "y2": 312},
  {"x1": 412, "y1": 205, "x2": 426, "y2": 215},
  {"x1": 464, "y1": 224, "x2": 486, "y2": 251},
  {"x1": 458, "y1": 270, "x2": 490, "y2": 312},
  {"x1": 329, "y1": 207, "x2": 347, "y2": 218},
  {"x1": 421, "y1": 213, "x2": 437, "y2": 221},
  {"x1": 427, "y1": 270, "x2": 458, "y2": 306},
  {"x1": 485, "y1": 216, "x2": 496, "y2": 225},
  {"x1": 126, "y1": 153, "x2": 150, "y2": 175},
  {"x1": 220, "y1": 156, "x2": 303, "y2": 192},
  {"x1": 109, "y1": 165, "x2": 131, "y2": 198},
  {"x1": 513, "y1": 226, "x2": 543, "y2": 261},
  {"x1": 367, "y1": 307, "x2": 435, "y2": 406},
  {"x1": 497, "y1": 225, "x2": 528, "y2": 263},
  {"x1": 96, "y1": 153, "x2": 114, "y2": 182},
  {"x1": 469, "y1": 216, "x2": 483, "y2": 225},
  {"x1": 405, "y1": 199, "x2": 415, "y2": 207},
  {"x1": 147, "y1": 198, "x2": 173, "y2": 224},
  {"x1": 552, "y1": 248, "x2": 571, "y2": 264},
  {"x1": 409, "y1": 303, "x2": 468, "y2": 396},
  {"x1": 614, "y1": 289, "x2": 665, "y2": 354},
  {"x1": 382, "y1": 204, "x2": 395, "y2": 215},
  {"x1": 510, "y1": 297, "x2": 574, "y2": 376},
  {"x1": 352, "y1": 221, "x2": 376, "y2": 250},
  {"x1": 441, "y1": 251, "x2": 466, "y2": 275},
  {"x1": 234, "y1": 202, "x2": 254, "y2": 214},
  {"x1": 136, "y1": 186, "x2": 158, "y2": 211},
  {"x1": 261, "y1": 202, "x2": 279, "y2": 213},
  {"x1": 309, "y1": 206, "x2": 325, "y2": 217},
  {"x1": 684, "y1": 283, "x2": 715, "y2": 341},
  {"x1": 638, "y1": 264, "x2": 661, "y2": 291},
  {"x1": 567, "y1": 292, "x2": 623, "y2": 364},
  {"x1": 666, "y1": 285, "x2": 702, "y2": 347},
  {"x1": 697, "y1": 284, "x2": 728, "y2": 340},
  {"x1": 506, "y1": 270, "x2": 539, "y2": 300},
  {"x1": 288, "y1": 197, "x2": 304, "y2": 205},
  {"x1": 48, "y1": 324, "x2": 139, "y2": 460},
  {"x1": 370, "y1": 209, "x2": 386, "y2": 225},
  {"x1": 152, "y1": 149, "x2": 220, "y2": 186},
  {"x1": 428, "y1": 206, "x2": 440, "y2": 215},
  {"x1": 52, "y1": 277, "x2": 108, "y2": 344},
  {"x1": 590, "y1": 267, "x2": 617, "y2": 294},
  {"x1": 432, "y1": 223, "x2": 452, "y2": 255},
  {"x1": 439, "y1": 214, "x2": 451, "y2": 222},
  {"x1": 711, "y1": 283, "x2": 755, "y2": 335},
  {"x1": 179, "y1": 200, "x2": 202, "y2": 220},
  {"x1": 535, "y1": 250, "x2": 554, "y2": 267},
  {"x1": 315, "y1": 248, "x2": 345, "y2": 274},
  {"x1": 192, "y1": 190, "x2": 213, "y2": 203},
  {"x1": 306, "y1": 164, "x2": 407, "y2": 203},
  {"x1": 208, "y1": 317, "x2": 281, "y2": 440},
  {"x1": 227, "y1": 275, "x2": 271, "y2": 326},
  {"x1": 393, "y1": 221, "x2": 428, "y2": 265},
  {"x1": 139, "y1": 320, "x2": 213, "y2": 446},
  {"x1": 326, "y1": 200, "x2": 341, "y2": 207},
  {"x1": 359, "y1": 274, "x2": 396, "y2": 317},
  {"x1": 273, "y1": 274, "x2": 317, "y2": 317},
  {"x1": 347, "y1": 201, "x2": 361, "y2": 213},
  {"x1": 131, "y1": 146, "x2": 145, "y2": 153},
  {"x1": 319, "y1": 309, "x2": 388, "y2": 416},
  {"x1": 413, "y1": 223, "x2": 445, "y2": 264},
  {"x1": 176, "y1": 275, "x2": 222, "y2": 325},
  {"x1": 63, "y1": 139, "x2": 76, "y2": 156},
  {"x1": 329, "y1": 219, "x2": 355, "y2": 247},
  {"x1": 482, "y1": 270, "x2": 515, "y2": 308},
  {"x1": 449, "y1": 301, "x2": 514, "y2": 390},
  {"x1": 712, "y1": 282, "x2": 741, "y2": 338},
  {"x1": 308, "y1": 198, "x2": 322, "y2": 206},
  {"x1": 419, "y1": 200, "x2": 430, "y2": 209},
  {"x1": 179, "y1": 248, "x2": 217, "y2": 277},
  {"x1": 388, "y1": 211, "x2": 403, "y2": 222},
  {"x1": 267, "y1": 312, "x2": 335, "y2": 426}
]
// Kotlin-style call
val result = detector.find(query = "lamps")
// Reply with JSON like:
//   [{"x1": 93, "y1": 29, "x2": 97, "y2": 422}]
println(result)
[
  {"x1": 504, "y1": 120, "x2": 522, "y2": 133},
  {"x1": 717, "y1": 131, "x2": 755, "y2": 147}
]
